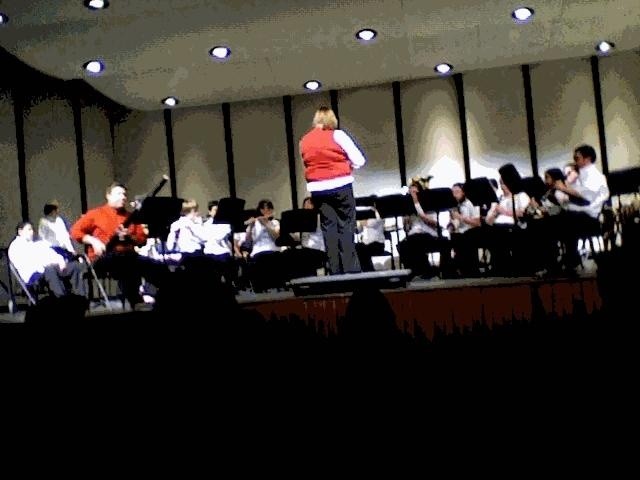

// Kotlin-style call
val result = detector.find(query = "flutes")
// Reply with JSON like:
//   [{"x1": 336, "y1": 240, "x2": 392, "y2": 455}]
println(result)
[{"x1": 243, "y1": 208, "x2": 288, "y2": 226}]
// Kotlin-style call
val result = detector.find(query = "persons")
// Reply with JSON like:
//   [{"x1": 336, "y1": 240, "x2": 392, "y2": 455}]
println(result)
[{"x1": 298, "y1": 105, "x2": 366, "y2": 275}]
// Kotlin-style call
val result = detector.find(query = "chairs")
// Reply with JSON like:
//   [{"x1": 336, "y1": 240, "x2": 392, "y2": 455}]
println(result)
[{"x1": 4, "y1": 247, "x2": 128, "y2": 315}]
